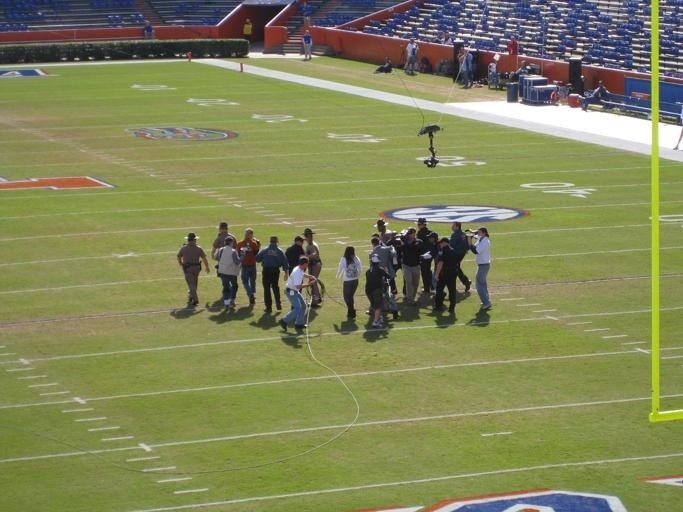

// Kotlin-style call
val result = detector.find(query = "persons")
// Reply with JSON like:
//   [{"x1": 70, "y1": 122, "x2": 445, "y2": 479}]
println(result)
[
  {"x1": 673, "y1": 107, "x2": 683, "y2": 151},
  {"x1": 211, "y1": 222, "x2": 323, "y2": 335},
  {"x1": 243, "y1": 19, "x2": 254, "y2": 42},
  {"x1": 365, "y1": 220, "x2": 492, "y2": 331},
  {"x1": 580, "y1": 81, "x2": 610, "y2": 112},
  {"x1": 371, "y1": 57, "x2": 392, "y2": 73},
  {"x1": 441, "y1": 31, "x2": 476, "y2": 88},
  {"x1": 404, "y1": 37, "x2": 420, "y2": 76},
  {"x1": 301, "y1": 29, "x2": 314, "y2": 62},
  {"x1": 177, "y1": 233, "x2": 209, "y2": 304},
  {"x1": 507, "y1": 34, "x2": 519, "y2": 55},
  {"x1": 510, "y1": 60, "x2": 530, "y2": 81},
  {"x1": 334, "y1": 246, "x2": 360, "y2": 318},
  {"x1": 142, "y1": 20, "x2": 156, "y2": 40}
]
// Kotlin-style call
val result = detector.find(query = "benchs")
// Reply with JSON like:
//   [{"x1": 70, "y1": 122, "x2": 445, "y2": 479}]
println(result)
[
  {"x1": 314, "y1": 1, "x2": 682, "y2": 79},
  {"x1": 281, "y1": 1, "x2": 323, "y2": 38},
  {"x1": 0, "y1": 0, "x2": 234, "y2": 32},
  {"x1": 585, "y1": 88, "x2": 682, "y2": 125}
]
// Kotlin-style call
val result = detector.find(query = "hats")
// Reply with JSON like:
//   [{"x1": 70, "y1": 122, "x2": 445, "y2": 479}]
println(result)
[
  {"x1": 437, "y1": 236, "x2": 449, "y2": 244},
  {"x1": 294, "y1": 235, "x2": 307, "y2": 242},
  {"x1": 296, "y1": 256, "x2": 310, "y2": 266},
  {"x1": 269, "y1": 235, "x2": 279, "y2": 244},
  {"x1": 414, "y1": 217, "x2": 430, "y2": 226},
  {"x1": 301, "y1": 227, "x2": 315, "y2": 236},
  {"x1": 407, "y1": 227, "x2": 416, "y2": 235},
  {"x1": 373, "y1": 218, "x2": 389, "y2": 228},
  {"x1": 371, "y1": 253, "x2": 382, "y2": 263},
  {"x1": 183, "y1": 232, "x2": 200, "y2": 240},
  {"x1": 425, "y1": 231, "x2": 438, "y2": 241}
]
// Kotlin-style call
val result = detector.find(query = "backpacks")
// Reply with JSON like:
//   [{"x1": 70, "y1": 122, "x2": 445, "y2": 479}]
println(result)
[{"x1": 346, "y1": 263, "x2": 358, "y2": 278}]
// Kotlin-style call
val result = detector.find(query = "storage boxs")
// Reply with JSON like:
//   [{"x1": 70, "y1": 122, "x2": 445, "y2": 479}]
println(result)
[{"x1": 518, "y1": 74, "x2": 557, "y2": 103}]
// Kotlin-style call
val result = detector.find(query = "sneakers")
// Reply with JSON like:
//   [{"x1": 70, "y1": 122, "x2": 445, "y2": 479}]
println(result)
[
  {"x1": 278, "y1": 317, "x2": 288, "y2": 331},
  {"x1": 294, "y1": 323, "x2": 309, "y2": 329},
  {"x1": 447, "y1": 304, "x2": 455, "y2": 312},
  {"x1": 480, "y1": 300, "x2": 492, "y2": 310},
  {"x1": 263, "y1": 304, "x2": 273, "y2": 313},
  {"x1": 186, "y1": 297, "x2": 199, "y2": 305},
  {"x1": 249, "y1": 294, "x2": 256, "y2": 305},
  {"x1": 309, "y1": 298, "x2": 322, "y2": 307},
  {"x1": 465, "y1": 279, "x2": 472, "y2": 291},
  {"x1": 275, "y1": 303, "x2": 282, "y2": 310},
  {"x1": 372, "y1": 316, "x2": 389, "y2": 330},
  {"x1": 392, "y1": 309, "x2": 399, "y2": 320}
]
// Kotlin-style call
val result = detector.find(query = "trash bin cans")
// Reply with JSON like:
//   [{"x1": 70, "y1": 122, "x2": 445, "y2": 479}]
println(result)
[{"x1": 506, "y1": 83, "x2": 519, "y2": 103}]
